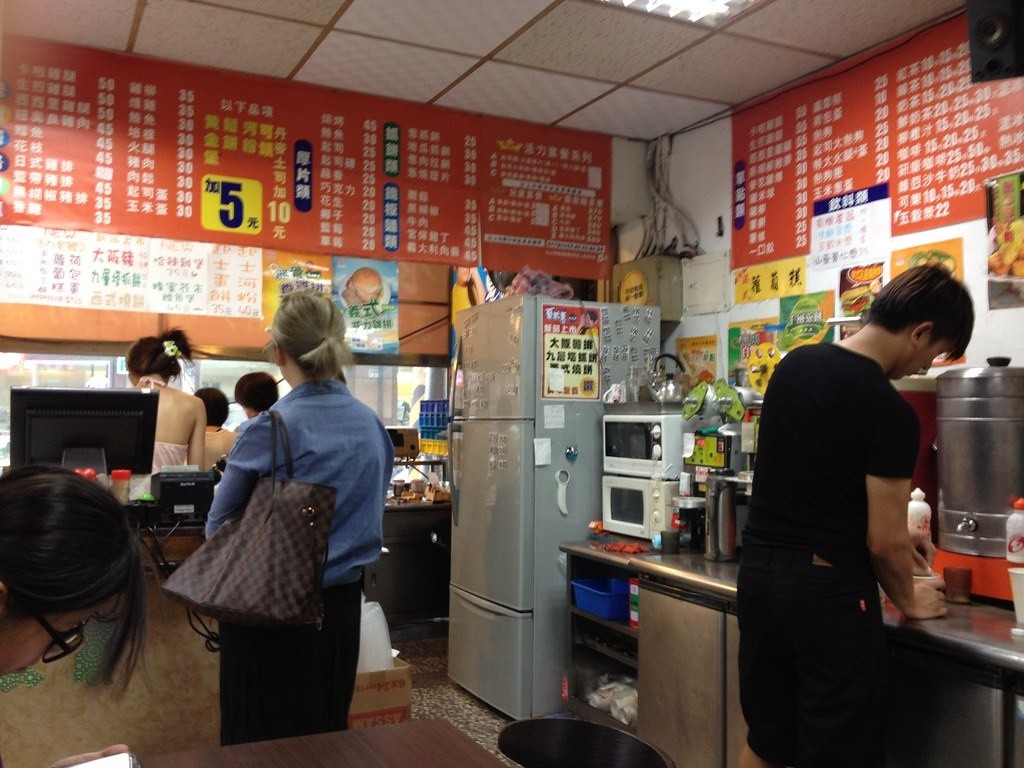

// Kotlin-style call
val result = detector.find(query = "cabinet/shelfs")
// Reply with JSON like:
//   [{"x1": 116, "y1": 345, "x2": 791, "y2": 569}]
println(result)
[{"x1": 136, "y1": 482, "x2": 452, "y2": 643}]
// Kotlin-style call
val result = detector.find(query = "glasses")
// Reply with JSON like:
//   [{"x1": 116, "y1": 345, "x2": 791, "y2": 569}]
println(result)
[{"x1": 4, "y1": 585, "x2": 94, "y2": 663}]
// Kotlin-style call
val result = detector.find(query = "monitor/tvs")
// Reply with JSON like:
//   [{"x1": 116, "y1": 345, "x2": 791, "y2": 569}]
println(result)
[{"x1": 10, "y1": 387, "x2": 159, "y2": 479}]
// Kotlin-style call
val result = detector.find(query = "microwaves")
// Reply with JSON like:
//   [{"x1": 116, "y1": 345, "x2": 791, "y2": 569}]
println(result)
[
  {"x1": 603, "y1": 411, "x2": 685, "y2": 480},
  {"x1": 602, "y1": 475, "x2": 680, "y2": 540}
]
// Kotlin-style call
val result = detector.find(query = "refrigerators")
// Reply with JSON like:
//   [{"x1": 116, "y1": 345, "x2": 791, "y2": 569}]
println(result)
[{"x1": 446, "y1": 293, "x2": 662, "y2": 721}]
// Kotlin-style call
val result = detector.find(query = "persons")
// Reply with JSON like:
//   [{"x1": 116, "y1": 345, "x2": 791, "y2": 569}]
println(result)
[
  {"x1": 0, "y1": 465, "x2": 150, "y2": 768},
  {"x1": 738, "y1": 263, "x2": 975, "y2": 768},
  {"x1": 205, "y1": 294, "x2": 395, "y2": 746},
  {"x1": 126, "y1": 330, "x2": 207, "y2": 500},
  {"x1": 235, "y1": 373, "x2": 279, "y2": 432},
  {"x1": 194, "y1": 387, "x2": 237, "y2": 471}
]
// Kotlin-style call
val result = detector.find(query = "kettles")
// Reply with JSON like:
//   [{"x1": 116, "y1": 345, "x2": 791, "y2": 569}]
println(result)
[{"x1": 645, "y1": 354, "x2": 689, "y2": 403}]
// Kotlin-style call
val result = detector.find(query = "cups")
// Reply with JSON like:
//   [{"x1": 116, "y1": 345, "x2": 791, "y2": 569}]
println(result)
[
  {"x1": 1007, "y1": 566, "x2": 1024, "y2": 623},
  {"x1": 914, "y1": 572, "x2": 941, "y2": 588},
  {"x1": 602, "y1": 383, "x2": 623, "y2": 404},
  {"x1": 660, "y1": 529, "x2": 680, "y2": 554}
]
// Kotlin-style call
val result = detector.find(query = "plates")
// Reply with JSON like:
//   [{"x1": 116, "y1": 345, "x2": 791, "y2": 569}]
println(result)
[{"x1": 339, "y1": 280, "x2": 391, "y2": 316}]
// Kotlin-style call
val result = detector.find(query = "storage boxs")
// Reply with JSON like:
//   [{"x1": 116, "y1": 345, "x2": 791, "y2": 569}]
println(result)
[
  {"x1": 348, "y1": 657, "x2": 412, "y2": 731},
  {"x1": 418, "y1": 399, "x2": 454, "y2": 455}
]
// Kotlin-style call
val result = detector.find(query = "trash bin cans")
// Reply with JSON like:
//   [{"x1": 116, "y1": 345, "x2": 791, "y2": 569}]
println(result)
[{"x1": 497, "y1": 717, "x2": 676, "y2": 768}]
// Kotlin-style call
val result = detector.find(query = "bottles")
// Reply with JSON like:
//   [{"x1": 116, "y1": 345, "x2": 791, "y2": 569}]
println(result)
[
  {"x1": 1005, "y1": 497, "x2": 1024, "y2": 563},
  {"x1": 111, "y1": 470, "x2": 131, "y2": 505},
  {"x1": 671, "y1": 496, "x2": 705, "y2": 551},
  {"x1": 626, "y1": 366, "x2": 640, "y2": 402},
  {"x1": 392, "y1": 479, "x2": 405, "y2": 496},
  {"x1": 907, "y1": 487, "x2": 931, "y2": 536}
]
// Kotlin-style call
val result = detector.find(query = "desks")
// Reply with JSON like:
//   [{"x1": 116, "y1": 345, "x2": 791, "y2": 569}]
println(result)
[{"x1": 136, "y1": 719, "x2": 509, "y2": 768}]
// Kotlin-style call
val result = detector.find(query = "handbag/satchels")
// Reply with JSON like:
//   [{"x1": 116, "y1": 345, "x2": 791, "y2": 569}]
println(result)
[{"x1": 160, "y1": 408, "x2": 338, "y2": 631}]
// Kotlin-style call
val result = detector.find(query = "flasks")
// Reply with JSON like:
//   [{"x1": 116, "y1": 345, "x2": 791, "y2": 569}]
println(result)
[{"x1": 704, "y1": 467, "x2": 734, "y2": 561}]
